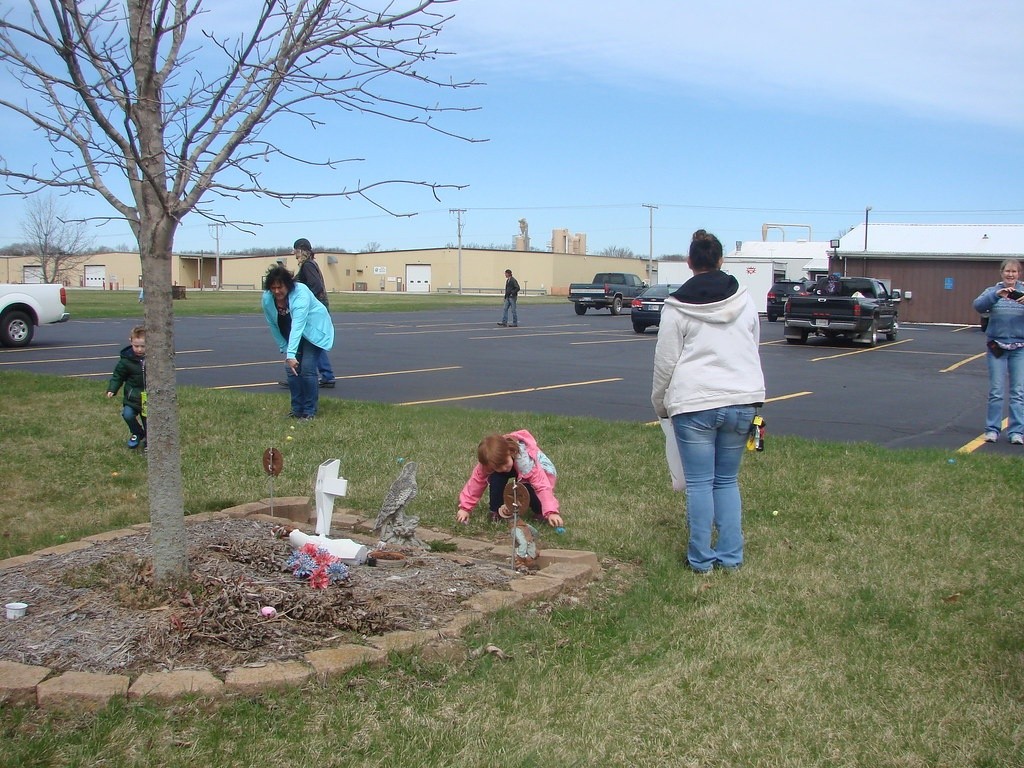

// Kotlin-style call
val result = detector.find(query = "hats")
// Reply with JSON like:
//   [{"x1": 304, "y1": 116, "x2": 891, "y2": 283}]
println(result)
[{"x1": 293, "y1": 238, "x2": 311, "y2": 250}]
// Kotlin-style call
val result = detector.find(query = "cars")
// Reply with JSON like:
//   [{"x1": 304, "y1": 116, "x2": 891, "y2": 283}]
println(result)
[{"x1": 631, "y1": 284, "x2": 682, "y2": 333}]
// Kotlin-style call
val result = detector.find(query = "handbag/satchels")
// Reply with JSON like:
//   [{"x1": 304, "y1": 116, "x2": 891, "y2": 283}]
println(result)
[{"x1": 658, "y1": 415, "x2": 687, "y2": 492}]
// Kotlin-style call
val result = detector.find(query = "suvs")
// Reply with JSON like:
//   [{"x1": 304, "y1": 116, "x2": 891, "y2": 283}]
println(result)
[{"x1": 767, "y1": 279, "x2": 816, "y2": 322}]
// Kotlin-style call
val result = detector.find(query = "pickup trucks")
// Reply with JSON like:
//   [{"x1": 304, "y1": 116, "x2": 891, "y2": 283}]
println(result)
[
  {"x1": 567, "y1": 272, "x2": 649, "y2": 316},
  {"x1": 0, "y1": 284, "x2": 70, "y2": 347},
  {"x1": 784, "y1": 276, "x2": 902, "y2": 348}
]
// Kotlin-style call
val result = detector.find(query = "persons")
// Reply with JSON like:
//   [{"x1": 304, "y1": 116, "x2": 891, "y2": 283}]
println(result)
[
  {"x1": 497, "y1": 269, "x2": 520, "y2": 327},
  {"x1": 651, "y1": 230, "x2": 765, "y2": 572},
  {"x1": 278, "y1": 238, "x2": 335, "y2": 388},
  {"x1": 261, "y1": 267, "x2": 334, "y2": 420},
  {"x1": 497, "y1": 506, "x2": 540, "y2": 571},
  {"x1": 108, "y1": 325, "x2": 148, "y2": 452},
  {"x1": 139, "y1": 289, "x2": 143, "y2": 303},
  {"x1": 457, "y1": 429, "x2": 564, "y2": 528},
  {"x1": 973, "y1": 258, "x2": 1024, "y2": 444}
]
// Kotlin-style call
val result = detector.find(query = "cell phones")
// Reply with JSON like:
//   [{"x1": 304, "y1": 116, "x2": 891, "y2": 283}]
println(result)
[{"x1": 1007, "y1": 290, "x2": 1024, "y2": 301}]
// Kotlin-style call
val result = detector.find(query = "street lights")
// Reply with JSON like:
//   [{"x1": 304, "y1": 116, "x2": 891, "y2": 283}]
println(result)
[{"x1": 863, "y1": 207, "x2": 872, "y2": 276}]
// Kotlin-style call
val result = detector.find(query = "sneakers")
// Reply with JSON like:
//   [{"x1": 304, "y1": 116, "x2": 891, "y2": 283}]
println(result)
[
  {"x1": 985, "y1": 430, "x2": 997, "y2": 443},
  {"x1": 1008, "y1": 431, "x2": 1024, "y2": 445}
]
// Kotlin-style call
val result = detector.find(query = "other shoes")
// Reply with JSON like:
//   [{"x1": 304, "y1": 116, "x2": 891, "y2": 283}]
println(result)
[
  {"x1": 287, "y1": 413, "x2": 298, "y2": 418},
  {"x1": 142, "y1": 437, "x2": 147, "y2": 451},
  {"x1": 488, "y1": 509, "x2": 502, "y2": 522},
  {"x1": 278, "y1": 379, "x2": 289, "y2": 386},
  {"x1": 497, "y1": 322, "x2": 507, "y2": 327},
  {"x1": 509, "y1": 324, "x2": 517, "y2": 327},
  {"x1": 531, "y1": 512, "x2": 545, "y2": 524},
  {"x1": 715, "y1": 562, "x2": 741, "y2": 574},
  {"x1": 318, "y1": 378, "x2": 335, "y2": 388},
  {"x1": 300, "y1": 415, "x2": 314, "y2": 422},
  {"x1": 127, "y1": 429, "x2": 145, "y2": 447},
  {"x1": 684, "y1": 556, "x2": 713, "y2": 577}
]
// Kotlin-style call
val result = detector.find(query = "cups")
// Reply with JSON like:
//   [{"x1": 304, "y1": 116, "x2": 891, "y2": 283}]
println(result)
[{"x1": 5, "y1": 603, "x2": 27, "y2": 619}]
